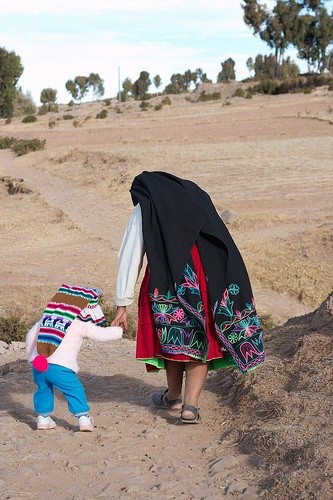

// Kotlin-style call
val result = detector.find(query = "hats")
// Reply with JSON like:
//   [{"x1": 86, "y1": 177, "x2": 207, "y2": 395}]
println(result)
[{"x1": 32, "y1": 283, "x2": 106, "y2": 372}]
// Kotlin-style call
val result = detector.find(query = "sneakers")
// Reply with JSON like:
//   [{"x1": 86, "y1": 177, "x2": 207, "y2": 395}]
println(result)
[
  {"x1": 37, "y1": 415, "x2": 57, "y2": 430},
  {"x1": 78, "y1": 416, "x2": 95, "y2": 432}
]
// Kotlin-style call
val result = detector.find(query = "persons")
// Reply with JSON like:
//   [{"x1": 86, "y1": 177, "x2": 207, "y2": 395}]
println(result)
[
  {"x1": 111, "y1": 171, "x2": 266, "y2": 423},
  {"x1": 26, "y1": 285, "x2": 126, "y2": 432}
]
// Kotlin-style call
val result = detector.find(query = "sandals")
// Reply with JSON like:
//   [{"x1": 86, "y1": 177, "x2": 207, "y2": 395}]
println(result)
[
  {"x1": 179, "y1": 405, "x2": 201, "y2": 424},
  {"x1": 151, "y1": 388, "x2": 182, "y2": 412}
]
